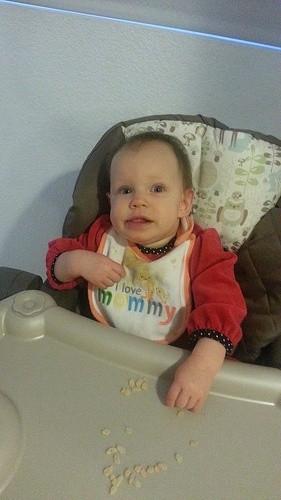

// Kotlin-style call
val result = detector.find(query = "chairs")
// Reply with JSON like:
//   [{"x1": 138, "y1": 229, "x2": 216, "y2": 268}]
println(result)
[{"x1": 0, "y1": 114, "x2": 281, "y2": 370}]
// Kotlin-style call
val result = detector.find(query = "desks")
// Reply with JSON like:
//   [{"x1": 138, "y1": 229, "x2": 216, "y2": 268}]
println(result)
[{"x1": 0, "y1": 289, "x2": 280, "y2": 500}]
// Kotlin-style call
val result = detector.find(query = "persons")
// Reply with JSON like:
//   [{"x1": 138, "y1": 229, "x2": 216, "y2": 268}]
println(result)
[{"x1": 44, "y1": 129, "x2": 248, "y2": 411}]
